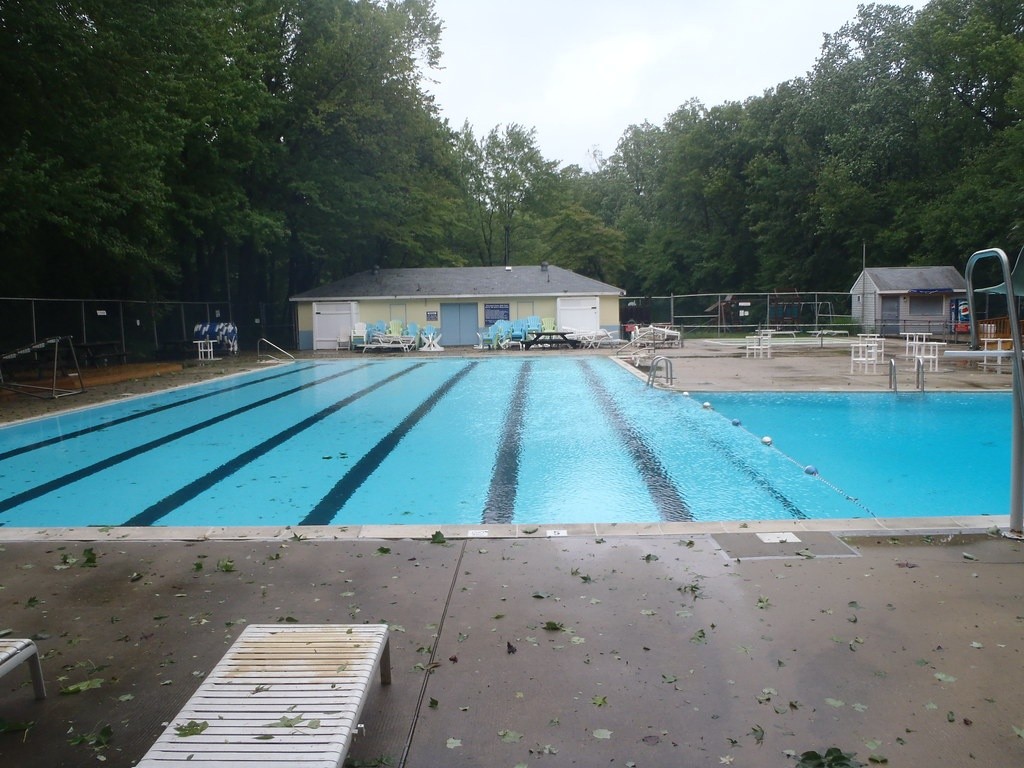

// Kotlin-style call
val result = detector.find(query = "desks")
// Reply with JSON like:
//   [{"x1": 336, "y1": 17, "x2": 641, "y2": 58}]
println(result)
[
  {"x1": 67, "y1": 341, "x2": 122, "y2": 369},
  {"x1": 526, "y1": 332, "x2": 575, "y2": 350}
]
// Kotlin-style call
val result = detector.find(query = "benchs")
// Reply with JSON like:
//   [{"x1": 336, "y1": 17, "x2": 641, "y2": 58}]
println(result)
[
  {"x1": 807, "y1": 331, "x2": 849, "y2": 338},
  {"x1": 755, "y1": 330, "x2": 800, "y2": 339},
  {"x1": 520, "y1": 339, "x2": 581, "y2": 349},
  {"x1": 83, "y1": 353, "x2": 128, "y2": 368}
]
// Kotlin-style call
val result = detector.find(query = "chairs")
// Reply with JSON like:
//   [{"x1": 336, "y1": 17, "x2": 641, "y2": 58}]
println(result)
[
  {"x1": 472, "y1": 317, "x2": 681, "y2": 351},
  {"x1": 133, "y1": 624, "x2": 391, "y2": 768},
  {"x1": 0, "y1": 638, "x2": 47, "y2": 701},
  {"x1": 337, "y1": 320, "x2": 444, "y2": 353}
]
